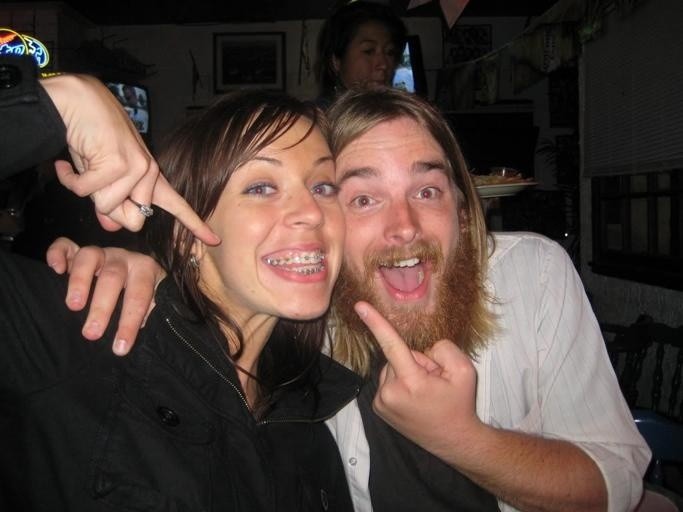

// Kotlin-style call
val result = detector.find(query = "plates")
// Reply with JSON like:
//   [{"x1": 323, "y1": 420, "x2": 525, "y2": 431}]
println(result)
[{"x1": 472, "y1": 181, "x2": 539, "y2": 198}]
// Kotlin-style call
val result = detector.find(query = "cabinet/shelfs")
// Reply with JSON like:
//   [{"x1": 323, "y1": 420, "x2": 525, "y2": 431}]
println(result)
[{"x1": 437, "y1": 100, "x2": 535, "y2": 230}]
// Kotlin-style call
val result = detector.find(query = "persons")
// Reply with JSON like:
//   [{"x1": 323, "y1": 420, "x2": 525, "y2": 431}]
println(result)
[
  {"x1": 122, "y1": 86, "x2": 148, "y2": 132},
  {"x1": 314, "y1": 0, "x2": 408, "y2": 108},
  {"x1": 1, "y1": 71, "x2": 502, "y2": 511},
  {"x1": 47, "y1": 82, "x2": 653, "y2": 511}
]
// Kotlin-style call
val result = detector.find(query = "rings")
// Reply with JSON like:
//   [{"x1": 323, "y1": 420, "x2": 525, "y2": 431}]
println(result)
[{"x1": 126, "y1": 195, "x2": 154, "y2": 218}]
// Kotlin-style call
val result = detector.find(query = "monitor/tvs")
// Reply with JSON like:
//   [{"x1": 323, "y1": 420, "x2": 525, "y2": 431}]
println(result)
[{"x1": 101, "y1": 78, "x2": 153, "y2": 149}]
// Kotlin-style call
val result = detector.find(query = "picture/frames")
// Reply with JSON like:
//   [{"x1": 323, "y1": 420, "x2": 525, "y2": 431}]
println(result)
[
  {"x1": 442, "y1": 24, "x2": 493, "y2": 67},
  {"x1": 213, "y1": 32, "x2": 287, "y2": 96}
]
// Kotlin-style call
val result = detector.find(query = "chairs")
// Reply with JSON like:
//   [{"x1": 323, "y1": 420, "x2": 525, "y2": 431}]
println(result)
[{"x1": 601, "y1": 313, "x2": 683, "y2": 512}]
